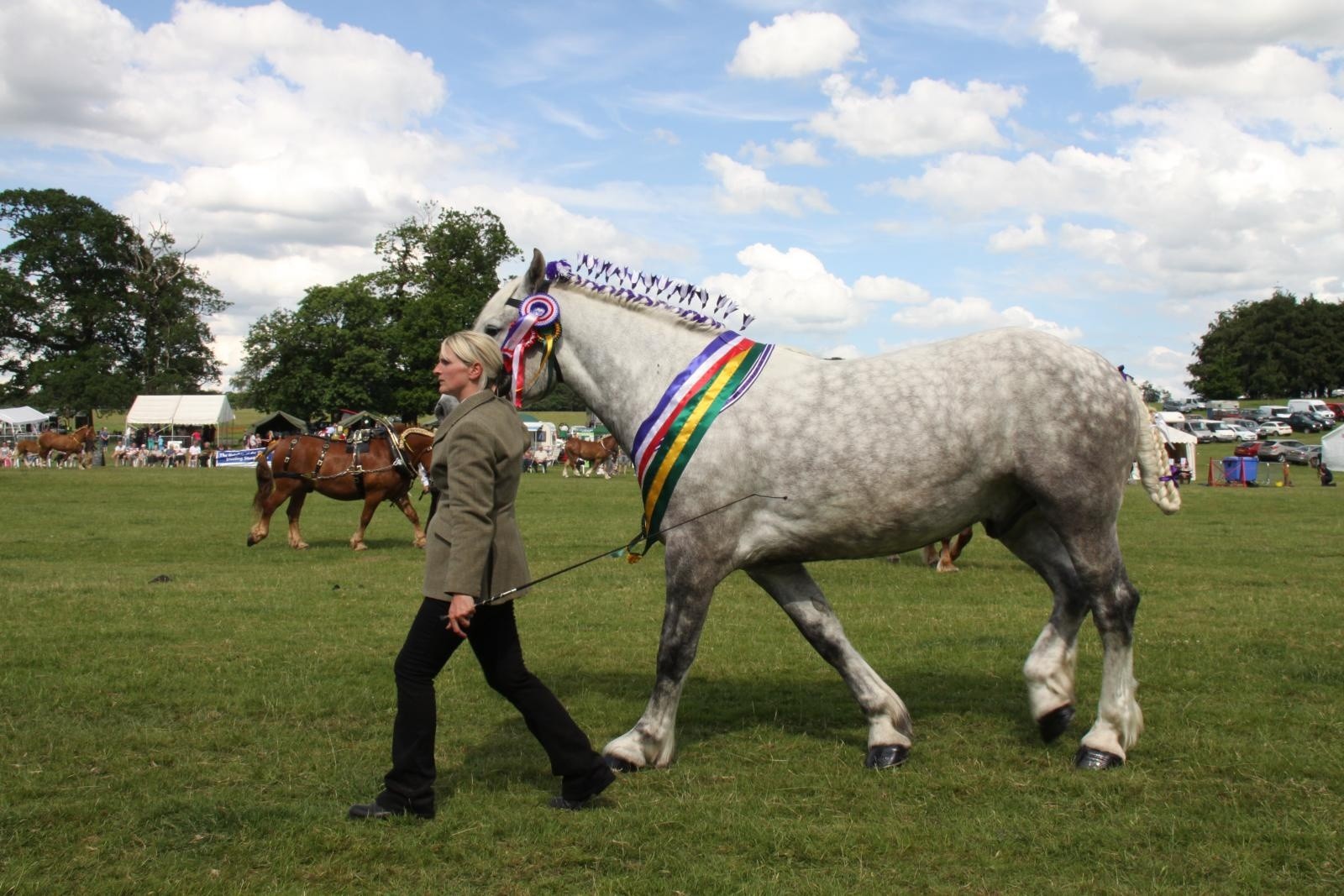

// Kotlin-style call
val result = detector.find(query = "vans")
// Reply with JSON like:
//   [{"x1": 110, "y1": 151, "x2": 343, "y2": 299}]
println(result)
[
  {"x1": 1288, "y1": 399, "x2": 1336, "y2": 422},
  {"x1": 1259, "y1": 406, "x2": 1292, "y2": 419},
  {"x1": 1185, "y1": 396, "x2": 1207, "y2": 410}
]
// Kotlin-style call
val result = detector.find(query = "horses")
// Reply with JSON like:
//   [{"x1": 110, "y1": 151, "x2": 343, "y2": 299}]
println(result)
[
  {"x1": 561, "y1": 430, "x2": 620, "y2": 479},
  {"x1": 13, "y1": 440, "x2": 53, "y2": 469},
  {"x1": 38, "y1": 424, "x2": 98, "y2": 470},
  {"x1": 249, "y1": 424, "x2": 439, "y2": 552},
  {"x1": 435, "y1": 248, "x2": 1182, "y2": 775},
  {"x1": 924, "y1": 522, "x2": 976, "y2": 573}
]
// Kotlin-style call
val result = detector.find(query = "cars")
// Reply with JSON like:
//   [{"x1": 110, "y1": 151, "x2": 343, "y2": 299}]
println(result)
[
  {"x1": 1228, "y1": 424, "x2": 1257, "y2": 443},
  {"x1": 1183, "y1": 414, "x2": 1203, "y2": 420},
  {"x1": 1233, "y1": 441, "x2": 1266, "y2": 461},
  {"x1": 1259, "y1": 421, "x2": 1293, "y2": 437},
  {"x1": 1286, "y1": 444, "x2": 1323, "y2": 467}
]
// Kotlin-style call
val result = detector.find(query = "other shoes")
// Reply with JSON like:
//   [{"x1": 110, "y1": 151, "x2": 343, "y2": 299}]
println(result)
[
  {"x1": 550, "y1": 768, "x2": 616, "y2": 809},
  {"x1": 350, "y1": 802, "x2": 411, "y2": 820}
]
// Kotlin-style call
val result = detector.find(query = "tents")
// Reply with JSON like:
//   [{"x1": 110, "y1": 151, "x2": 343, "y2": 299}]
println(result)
[
  {"x1": 125, "y1": 394, "x2": 236, "y2": 449},
  {"x1": 0, "y1": 406, "x2": 50, "y2": 436},
  {"x1": 1321, "y1": 423, "x2": 1344, "y2": 472},
  {"x1": 253, "y1": 411, "x2": 307, "y2": 437},
  {"x1": 337, "y1": 410, "x2": 380, "y2": 429},
  {"x1": 1133, "y1": 422, "x2": 1197, "y2": 482}
]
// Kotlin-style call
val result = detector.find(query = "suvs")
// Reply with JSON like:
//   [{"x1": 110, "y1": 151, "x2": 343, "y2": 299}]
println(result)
[
  {"x1": 1162, "y1": 400, "x2": 1191, "y2": 413},
  {"x1": 1214, "y1": 409, "x2": 1243, "y2": 421},
  {"x1": 1257, "y1": 439, "x2": 1305, "y2": 463},
  {"x1": 1240, "y1": 407, "x2": 1274, "y2": 423},
  {"x1": 1327, "y1": 402, "x2": 1344, "y2": 421},
  {"x1": 1201, "y1": 419, "x2": 1234, "y2": 442},
  {"x1": 1234, "y1": 420, "x2": 1267, "y2": 439},
  {"x1": 1183, "y1": 421, "x2": 1214, "y2": 445},
  {"x1": 1293, "y1": 411, "x2": 1337, "y2": 431}
]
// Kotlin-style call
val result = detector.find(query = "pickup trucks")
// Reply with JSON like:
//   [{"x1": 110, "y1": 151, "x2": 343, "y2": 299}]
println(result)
[{"x1": 1270, "y1": 414, "x2": 1323, "y2": 435}]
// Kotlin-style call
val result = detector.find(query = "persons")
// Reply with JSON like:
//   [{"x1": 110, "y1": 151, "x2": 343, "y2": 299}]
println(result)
[
  {"x1": 1300, "y1": 386, "x2": 1329, "y2": 399},
  {"x1": 0, "y1": 427, "x2": 275, "y2": 468},
  {"x1": 350, "y1": 327, "x2": 617, "y2": 826},
  {"x1": 575, "y1": 446, "x2": 629, "y2": 476},
  {"x1": 1320, "y1": 463, "x2": 1336, "y2": 486},
  {"x1": 533, "y1": 446, "x2": 547, "y2": 473},
  {"x1": 418, "y1": 461, "x2": 440, "y2": 536},
  {"x1": 521, "y1": 447, "x2": 532, "y2": 473}
]
